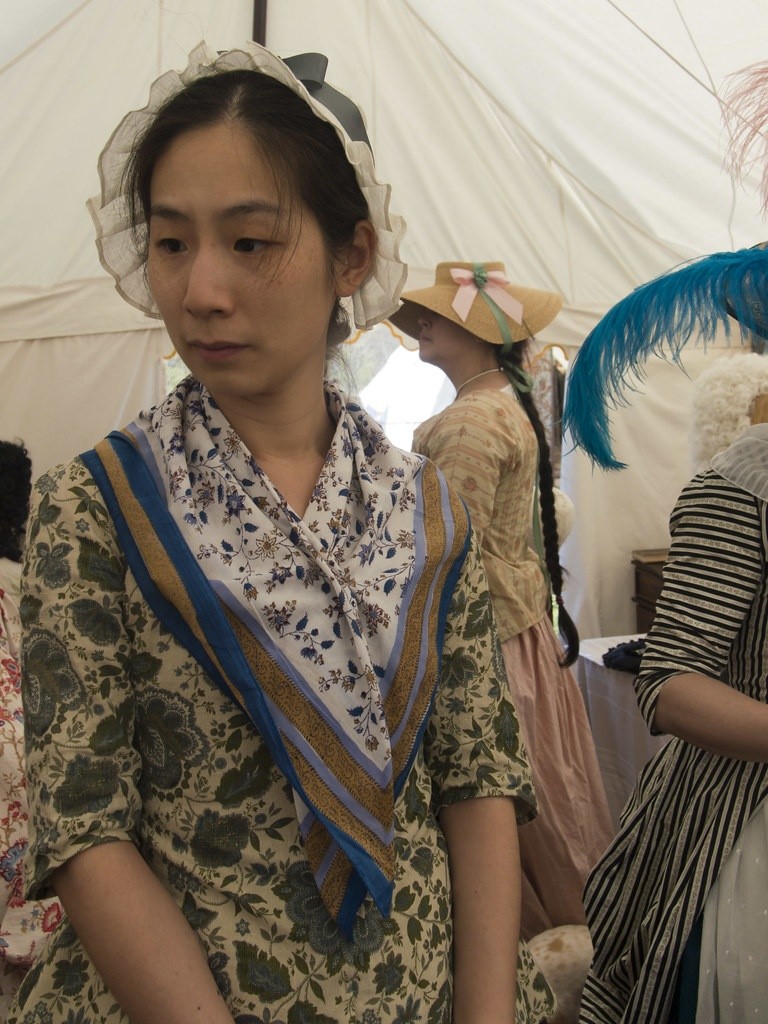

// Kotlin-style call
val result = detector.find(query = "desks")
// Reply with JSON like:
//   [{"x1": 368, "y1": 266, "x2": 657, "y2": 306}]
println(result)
[{"x1": 560, "y1": 631, "x2": 672, "y2": 830}]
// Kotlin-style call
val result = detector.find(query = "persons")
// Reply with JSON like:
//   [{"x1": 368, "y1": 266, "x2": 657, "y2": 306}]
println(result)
[
  {"x1": 579, "y1": 422, "x2": 767, "y2": 1023},
  {"x1": 19, "y1": 33, "x2": 540, "y2": 1024},
  {"x1": 0, "y1": 440, "x2": 66, "y2": 1024},
  {"x1": 377, "y1": 256, "x2": 616, "y2": 1023}
]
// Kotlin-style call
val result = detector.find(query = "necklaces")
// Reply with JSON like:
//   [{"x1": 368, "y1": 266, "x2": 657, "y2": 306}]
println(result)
[{"x1": 457, "y1": 365, "x2": 505, "y2": 393}]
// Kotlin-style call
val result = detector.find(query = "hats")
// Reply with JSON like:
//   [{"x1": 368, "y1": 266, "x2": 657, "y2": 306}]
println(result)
[
  {"x1": 86, "y1": 40, "x2": 408, "y2": 344},
  {"x1": 719, "y1": 241, "x2": 768, "y2": 340},
  {"x1": 388, "y1": 261, "x2": 564, "y2": 344}
]
